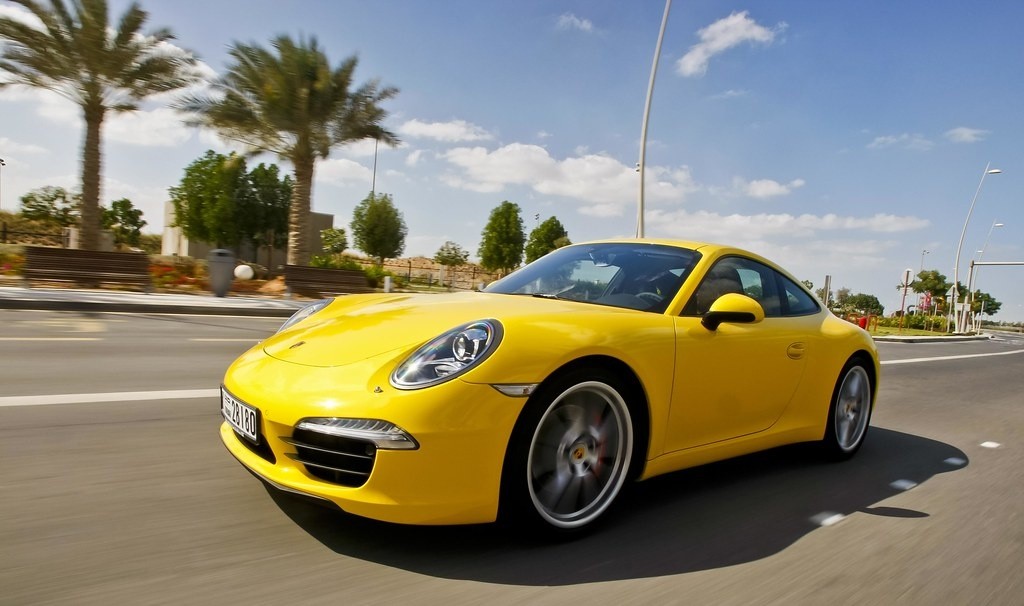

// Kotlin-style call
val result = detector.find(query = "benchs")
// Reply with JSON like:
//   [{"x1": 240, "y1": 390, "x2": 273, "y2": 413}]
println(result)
[
  {"x1": 19, "y1": 244, "x2": 153, "y2": 295},
  {"x1": 284, "y1": 263, "x2": 376, "y2": 300}
]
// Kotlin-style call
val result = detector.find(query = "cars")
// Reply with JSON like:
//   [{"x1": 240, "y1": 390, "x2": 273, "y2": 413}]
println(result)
[{"x1": 217, "y1": 237, "x2": 880, "y2": 542}]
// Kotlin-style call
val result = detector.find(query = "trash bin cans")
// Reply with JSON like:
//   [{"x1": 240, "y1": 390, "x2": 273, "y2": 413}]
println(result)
[{"x1": 208, "y1": 249, "x2": 234, "y2": 298}]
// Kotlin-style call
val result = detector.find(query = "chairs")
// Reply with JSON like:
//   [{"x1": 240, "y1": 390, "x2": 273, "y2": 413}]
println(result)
[{"x1": 710, "y1": 264, "x2": 743, "y2": 294}]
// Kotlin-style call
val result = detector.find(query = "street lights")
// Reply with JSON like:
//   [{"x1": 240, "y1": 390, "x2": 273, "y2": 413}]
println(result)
[
  {"x1": 951, "y1": 161, "x2": 1003, "y2": 336},
  {"x1": 913, "y1": 250, "x2": 929, "y2": 316},
  {"x1": 971, "y1": 219, "x2": 1004, "y2": 332}
]
